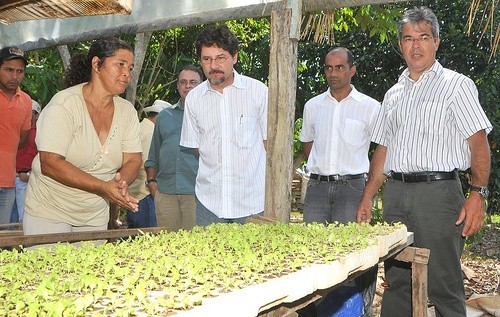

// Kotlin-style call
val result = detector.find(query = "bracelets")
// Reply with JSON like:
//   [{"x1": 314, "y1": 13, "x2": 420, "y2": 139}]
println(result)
[{"x1": 27, "y1": 172, "x2": 32, "y2": 176}]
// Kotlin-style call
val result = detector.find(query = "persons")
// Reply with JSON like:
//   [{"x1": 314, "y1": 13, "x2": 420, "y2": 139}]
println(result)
[
  {"x1": 22, "y1": 37, "x2": 143, "y2": 251},
  {"x1": 125, "y1": 99, "x2": 173, "y2": 228},
  {"x1": 17, "y1": 99, "x2": 41, "y2": 223},
  {"x1": 299, "y1": 47, "x2": 382, "y2": 317},
  {"x1": 357, "y1": 7, "x2": 494, "y2": 317},
  {"x1": 0, "y1": 45, "x2": 32, "y2": 233},
  {"x1": 180, "y1": 26, "x2": 268, "y2": 228},
  {"x1": 144, "y1": 65, "x2": 203, "y2": 233}
]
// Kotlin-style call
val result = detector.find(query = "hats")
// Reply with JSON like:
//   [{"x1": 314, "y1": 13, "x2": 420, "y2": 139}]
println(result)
[
  {"x1": 1, "y1": 45, "x2": 29, "y2": 66},
  {"x1": 142, "y1": 100, "x2": 173, "y2": 113},
  {"x1": 32, "y1": 100, "x2": 41, "y2": 113}
]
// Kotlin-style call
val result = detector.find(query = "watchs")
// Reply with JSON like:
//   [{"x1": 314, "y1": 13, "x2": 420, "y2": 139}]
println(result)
[
  {"x1": 145, "y1": 179, "x2": 158, "y2": 186},
  {"x1": 469, "y1": 184, "x2": 490, "y2": 199}
]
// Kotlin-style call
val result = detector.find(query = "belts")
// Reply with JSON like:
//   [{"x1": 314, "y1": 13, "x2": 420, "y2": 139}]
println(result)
[
  {"x1": 310, "y1": 172, "x2": 364, "y2": 182},
  {"x1": 391, "y1": 170, "x2": 456, "y2": 184}
]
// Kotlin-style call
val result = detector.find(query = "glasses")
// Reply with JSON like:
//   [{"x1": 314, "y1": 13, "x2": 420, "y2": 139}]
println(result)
[
  {"x1": 401, "y1": 34, "x2": 433, "y2": 44},
  {"x1": 178, "y1": 79, "x2": 200, "y2": 85},
  {"x1": 201, "y1": 53, "x2": 231, "y2": 64}
]
